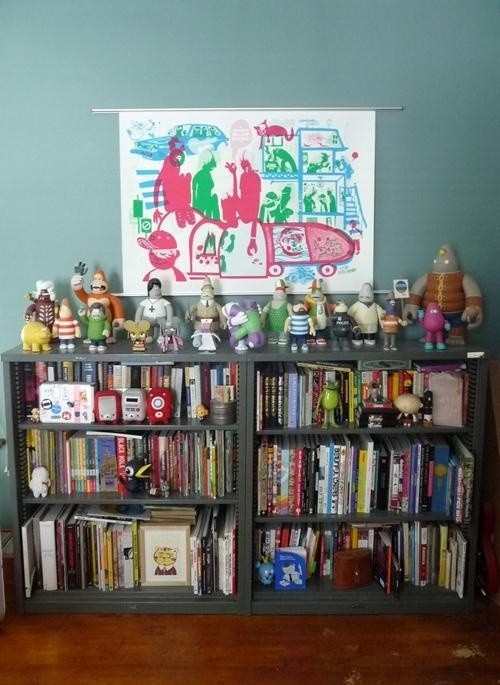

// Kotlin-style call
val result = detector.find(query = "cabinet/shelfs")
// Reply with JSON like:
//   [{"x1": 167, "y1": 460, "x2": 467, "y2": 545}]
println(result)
[
  {"x1": 0, "y1": 335, "x2": 247, "y2": 618},
  {"x1": 247, "y1": 339, "x2": 491, "y2": 616}
]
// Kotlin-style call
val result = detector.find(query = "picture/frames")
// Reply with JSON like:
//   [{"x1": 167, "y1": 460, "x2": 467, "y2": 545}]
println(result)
[{"x1": 134, "y1": 520, "x2": 194, "y2": 589}]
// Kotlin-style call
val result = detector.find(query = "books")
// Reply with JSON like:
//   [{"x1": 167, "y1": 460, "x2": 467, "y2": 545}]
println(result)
[
  {"x1": 256, "y1": 363, "x2": 474, "y2": 600},
  {"x1": 20, "y1": 361, "x2": 237, "y2": 601}
]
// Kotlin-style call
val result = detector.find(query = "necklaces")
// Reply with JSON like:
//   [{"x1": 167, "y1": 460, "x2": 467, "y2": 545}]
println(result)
[{"x1": 149, "y1": 298, "x2": 160, "y2": 312}]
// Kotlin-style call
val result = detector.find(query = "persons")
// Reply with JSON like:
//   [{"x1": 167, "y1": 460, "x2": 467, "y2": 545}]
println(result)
[
  {"x1": 222, "y1": 300, "x2": 264, "y2": 352},
  {"x1": 135, "y1": 278, "x2": 174, "y2": 344},
  {"x1": 18, "y1": 242, "x2": 484, "y2": 355},
  {"x1": 52, "y1": 300, "x2": 82, "y2": 349},
  {"x1": 184, "y1": 276, "x2": 225, "y2": 348},
  {"x1": 78, "y1": 304, "x2": 110, "y2": 351},
  {"x1": 261, "y1": 280, "x2": 385, "y2": 354}
]
